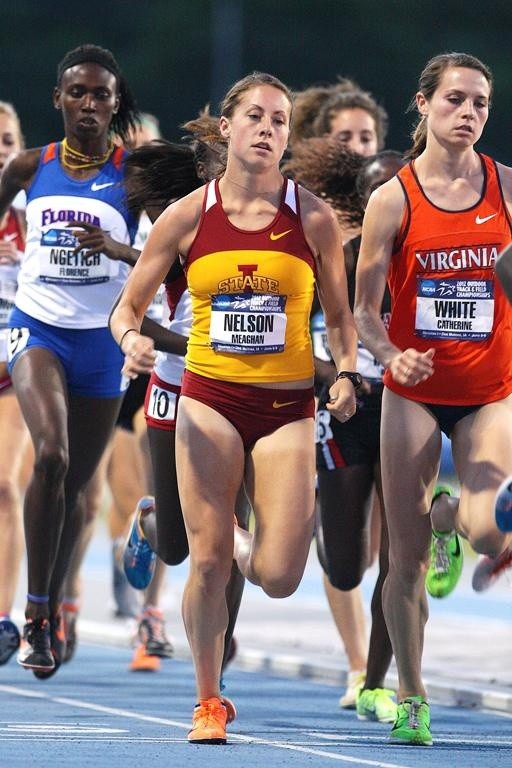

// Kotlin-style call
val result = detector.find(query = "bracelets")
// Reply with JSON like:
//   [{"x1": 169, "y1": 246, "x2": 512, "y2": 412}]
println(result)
[{"x1": 119, "y1": 329, "x2": 140, "y2": 357}]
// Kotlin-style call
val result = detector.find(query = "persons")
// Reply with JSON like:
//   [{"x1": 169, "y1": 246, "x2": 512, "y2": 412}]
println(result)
[
  {"x1": 58, "y1": 115, "x2": 175, "y2": 664},
  {"x1": 110, "y1": 72, "x2": 357, "y2": 744},
  {"x1": 472, "y1": 546, "x2": 510, "y2": 590},
  {"x1": 108, "y1": 465, "x2": 144, "y2": 621},
  {"x1": 2, "y1": 44, "x2": 185, "y2": 682},
  {"x1": 123, "y1": 114, "x2": 251, "y2": 725},
  {"x1": 279, "y1": 136, "x2": 413, "y2": 723},
  {"x1": 351, "y1": 53, "x2": 511, "y2": 745},
  {"x1": 493, "y1": 245, "x2": 512, "y2": 538},
  {"x1": 288, "y1": 80, "x2": 389, "y2": 710},
  {"x1": 1, "y1": 102, "x2": 28, "y2": 661}
]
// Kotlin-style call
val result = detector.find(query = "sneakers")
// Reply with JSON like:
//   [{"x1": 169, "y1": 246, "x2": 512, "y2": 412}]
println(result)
[
  {"x1": 130, "y1": 646, "x2": 158, "y2": 673},
  {"x1": 34, "y1": 599, "x2": 70, "y2": 681},
  {"x1": 123, "y1": 497, "x2": 157, "y2": 590},
  {"x1": 386, "y1": 696, "x2": 433, "y2": 745},
  {"x1": 0, "y1": 616, "x2": 22, "y2": 664},
  {"x1": 494, "y1": 477, "x2": 512, "y2": 533},
  {"x1": 65, "y1": 609, "x2": 85, "y2": 662},
  {"x1": 17, "y1": 619, "x2": 56, "y2": 670},
  {"x1": 338, "y1": 673, "x2": 370, "y2": 711},
  {"x1": 188, "y1": 697, "x2": 226, "y2": 744},
  {"x1": 427, "y1": 483, "x2": 463, "y2": 600},
  {"x1": 356, "y1": 686, "x2": 401, "y2": 722},
  {"x1": 139, "y1": 611, "x2": 175, "y2": 657},
  {"x1": 473, "y1": 546, "x2": 512, "y2": 594}
]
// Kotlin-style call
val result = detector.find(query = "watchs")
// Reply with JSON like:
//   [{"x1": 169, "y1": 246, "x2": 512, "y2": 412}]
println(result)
[{"x1": 336, "y1": 371, "x2": 361, "y2": 391}]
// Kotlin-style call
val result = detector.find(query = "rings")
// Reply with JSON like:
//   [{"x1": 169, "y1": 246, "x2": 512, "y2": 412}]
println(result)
[
  {"x1": 331, "y1": 397, "x2": 337, "y2": 400},
  {"x1": 345, "y1": 413, "x2": 349, "y2": 417},
  {"x1": 130, "y1": 351, "x2": 138, "y2": 358},
  {"x1": 404, "y1": 367, "x2": 409, "y2": 377}
]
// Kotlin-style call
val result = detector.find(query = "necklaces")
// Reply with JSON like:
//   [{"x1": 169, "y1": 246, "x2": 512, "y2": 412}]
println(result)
[
  {"x1": 223, "y1": 174, "x2": 283, "y2": 194},
  {"x1": 60, "y1": 137, "x2": 114, "y2": 171}
]
